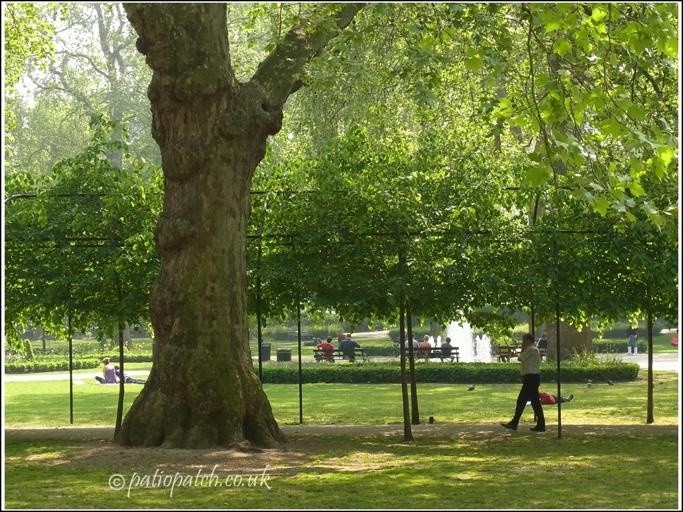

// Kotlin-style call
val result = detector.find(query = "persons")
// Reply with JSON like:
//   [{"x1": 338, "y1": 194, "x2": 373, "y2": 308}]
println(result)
[
  {"x1": 405, "y1": 333, "x2": 419, "y2": 356},
  {"x1": 536, "y1": 335, "x2": 546, "y2": 359},
  {"x1": 538, "y1": 391, "x2": 574, "y2": 405},
  {"x1": 501, "y1": 334, "x2": 546, "y2": 432},
  {"x1": 416, "y1": 334, "x2": 431, "y2": 357},
  {"x1": 339, "y1": 334, "x2": 360, "y2": 361},
  {"x1": 114, "y1": 365, "x2": 147, "y2": 384},
  {"x1": 95, "y1": 357, "x2": 118, "y2": 383},
  {"x1": 440, "y1": 337, "x2": 450, "y2": 361},
  {"x1": 316, "y1": 336, "x2": 335, "y2": 363},
  {"x1": 499, "y1": 331, "x2": 512, "y2": 362},
  {"x1": 627, "y1": 326, "x2": 639, "y2": 356}
]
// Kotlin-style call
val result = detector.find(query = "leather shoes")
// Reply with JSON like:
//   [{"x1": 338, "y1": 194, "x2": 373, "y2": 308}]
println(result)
[
  {"x1": 501, "y1": 423, "x2": 518, "y2": 430},
  {"x1": 530, "y1": 427, "x2": 545, "y2": 431}
]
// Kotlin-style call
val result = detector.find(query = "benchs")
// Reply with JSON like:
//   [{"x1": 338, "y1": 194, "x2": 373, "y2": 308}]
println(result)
[
  {"x1": 497, "y1": 346, "x2": 547, "y2": 362},
  {"x1": 288, "y1": 331, "x2": 312, "y2": 342},
  {"x1": 313, "y1": 348, "x2": 369, "y2": 363},
  {"x1": 405, "y1": 347, "x2": 460, "y2": 363}
]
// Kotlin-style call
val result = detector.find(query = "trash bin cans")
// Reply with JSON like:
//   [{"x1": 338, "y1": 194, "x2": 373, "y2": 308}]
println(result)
[
  {"x1": 276, "y1": 348, "x2": 292, "y2": 362},
  {"x1": 261, "y1": 343, "x2": 271, "y2": 361}
]
